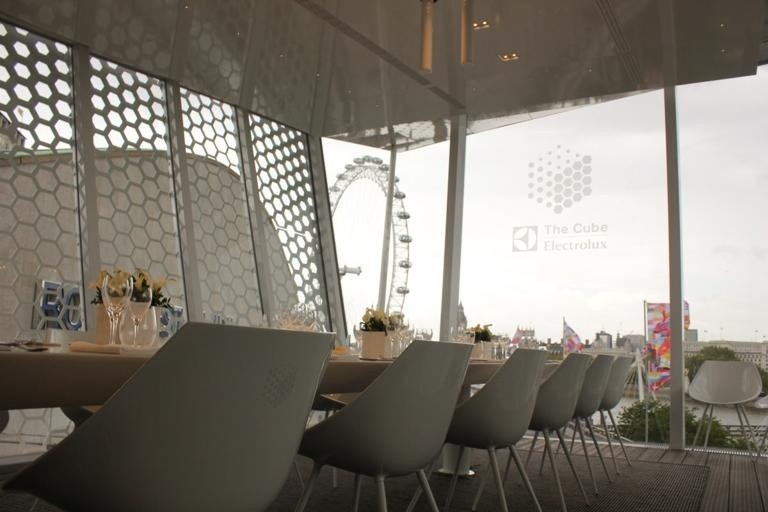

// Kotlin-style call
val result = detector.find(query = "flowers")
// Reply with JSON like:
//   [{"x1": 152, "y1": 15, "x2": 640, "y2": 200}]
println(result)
[{"x1": 88, "y1": 269, "x2": 171, "y2": 307}]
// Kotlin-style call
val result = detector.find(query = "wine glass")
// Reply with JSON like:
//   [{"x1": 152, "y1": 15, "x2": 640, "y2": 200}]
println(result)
[
  {"x1": 259, "y1": 301, "x2": 322, "y2": 332},
  {"x1": 353, "y1": 324, "x2": 365, "y2": 355},
  {"x1": 385, "y1": 319, "x2": 433, "y2": 359},
  {"x1": 100, "y1": 274, "x2": 152, "y2": 351},
  {"x1": 447, "y1": 321, "x2": 477, "y2": 357},
  {"x1": 487, "y1": 341, "x2": 518, "y2": 362}
]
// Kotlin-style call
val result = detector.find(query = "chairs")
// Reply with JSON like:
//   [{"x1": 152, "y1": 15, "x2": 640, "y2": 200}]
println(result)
[
  {"x1": 687, "y1": 357, "x2": 764, "y2": 459},
  {"x1": 0, "y1": 302, "x2": 633, "y2": 510}
]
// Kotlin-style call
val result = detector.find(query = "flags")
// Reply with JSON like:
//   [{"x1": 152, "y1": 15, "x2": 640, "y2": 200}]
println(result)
[
  {"x1": 510, "y1": 329, "x2": 522, "y2": 344},
  {"x1": 646, "y1": 301, "x2": 692, "y2": 390},
  {"x1": 564, "y1": 323, "x2": 584, "y2": 357}
]
